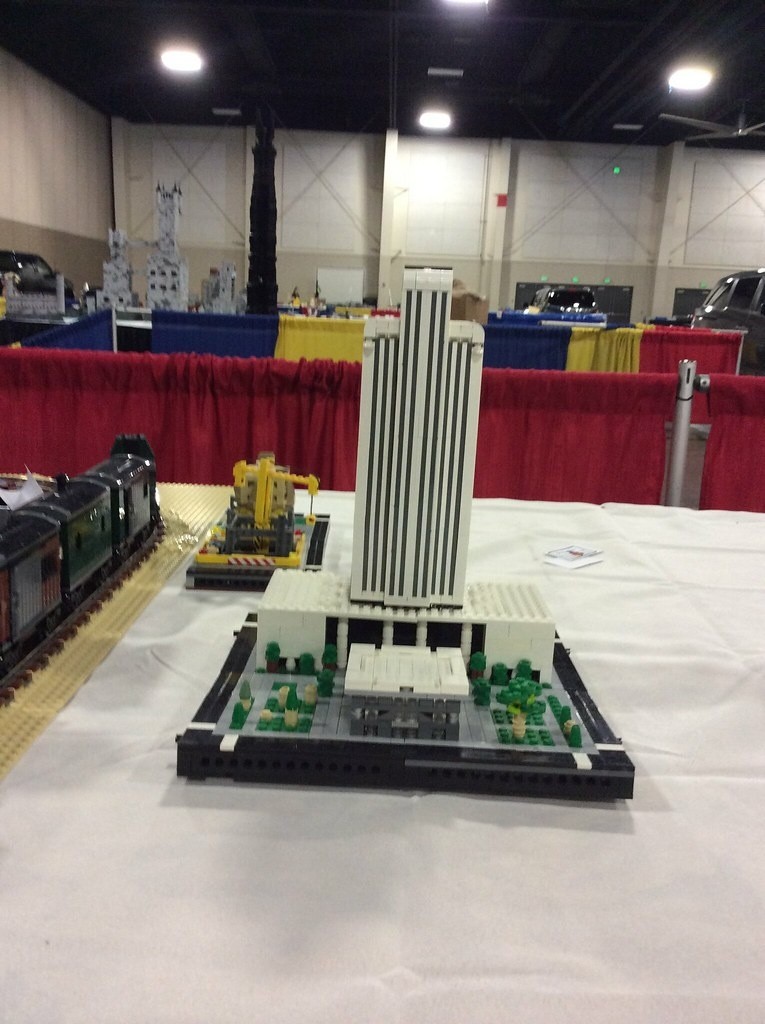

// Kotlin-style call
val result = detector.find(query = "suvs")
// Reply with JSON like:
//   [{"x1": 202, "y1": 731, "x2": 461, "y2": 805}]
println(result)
[
  {"x1": 0, "y1": 250, "x2": 80, "y2": 308},
  {"x1": 522, "y1": 282, "x2": 600, "y2": 315}
]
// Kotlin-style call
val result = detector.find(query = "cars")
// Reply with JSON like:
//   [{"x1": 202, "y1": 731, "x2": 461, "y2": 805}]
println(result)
[{"x1": 689, "y1": 268, "x2": 765, "y2": 378}]
